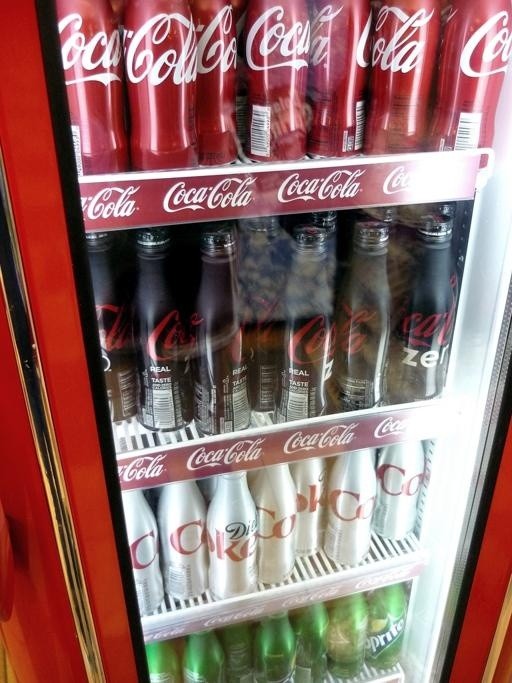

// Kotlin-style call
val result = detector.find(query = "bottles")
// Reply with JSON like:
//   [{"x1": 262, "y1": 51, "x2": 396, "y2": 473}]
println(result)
[
  {"x1": 144, "y1": 574, "x2": 407, "y2": 677},
  {"x1": 89, "y1": 204, "x2": 461, "y2": 441},
  {"x1": 120, "y1": 428, "x2": 430, "y2": 621},
  {"x1": 48, "y1": 0, "x2": 505, "y2": 170}
]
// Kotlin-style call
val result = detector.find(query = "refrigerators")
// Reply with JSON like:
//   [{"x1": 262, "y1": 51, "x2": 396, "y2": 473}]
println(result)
[{"x1": 5, "y1": 0, "x2": 510, "y2": 679}]
